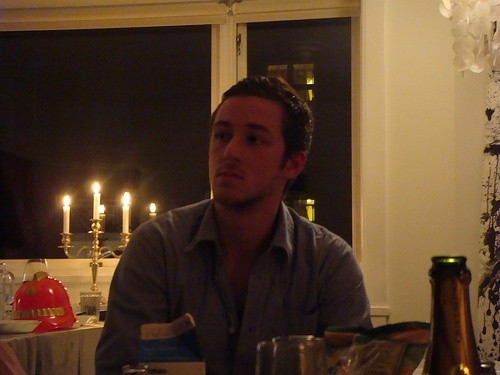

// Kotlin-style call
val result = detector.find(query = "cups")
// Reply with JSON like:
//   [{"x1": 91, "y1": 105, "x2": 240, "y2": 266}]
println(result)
[{"x1": 80, "y1": 292, "x2": 100, "y2": 323}]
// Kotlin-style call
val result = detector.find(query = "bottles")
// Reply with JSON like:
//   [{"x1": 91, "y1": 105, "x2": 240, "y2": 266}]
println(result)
[
  {"x1": 480, "y1": 362, "x2": 497, "y2": 375},
  {"x1": 0, "y1": 263, "x2": 15, "y2": 305},
  {"x1": 294, "y1": 198, "x2": 316, "y2": 222},
  {"x1": 257, "y1": 336, "x2": 326, "y2": 375},
  {"x1": 428, "y1": 256, "x2": 482, "y2": 375}
]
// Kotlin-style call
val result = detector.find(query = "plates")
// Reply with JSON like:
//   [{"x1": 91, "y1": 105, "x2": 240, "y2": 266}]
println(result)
[{"x1": 0, "y1": 320, "x2": 41, "y2": 332}]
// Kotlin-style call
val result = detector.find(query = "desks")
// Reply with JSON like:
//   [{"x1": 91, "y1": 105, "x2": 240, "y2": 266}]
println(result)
[{"x1": 0, "y1": 303, "x2": 108, "y2": 375}]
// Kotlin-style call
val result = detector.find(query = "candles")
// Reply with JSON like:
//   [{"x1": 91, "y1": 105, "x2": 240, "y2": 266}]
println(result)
[
  {"x1": 92, "y1": 182, "x2": 100, "y2": 219},
  {"x1": 122, "y1": 192, "x2": 130, "y2": 233},
  {"x1": 149, "y1": 203, "x2": 157, "y2": 219},
  {"x1": 63, "y1": 195, "x2": 70, "y2": 233},
  {"x1": 98, "y1": 205, "x2": 105, "y2": 239}
]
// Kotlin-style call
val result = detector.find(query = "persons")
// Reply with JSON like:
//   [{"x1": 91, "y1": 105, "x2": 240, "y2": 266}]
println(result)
[{"x1": 94, "y1": 75, "x2": 373, "y2": 375}]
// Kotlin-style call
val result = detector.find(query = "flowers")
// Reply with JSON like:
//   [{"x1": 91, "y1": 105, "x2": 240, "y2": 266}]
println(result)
[{"x1": 439, "y1": 0, "x2": 500, "y2": 80}]
